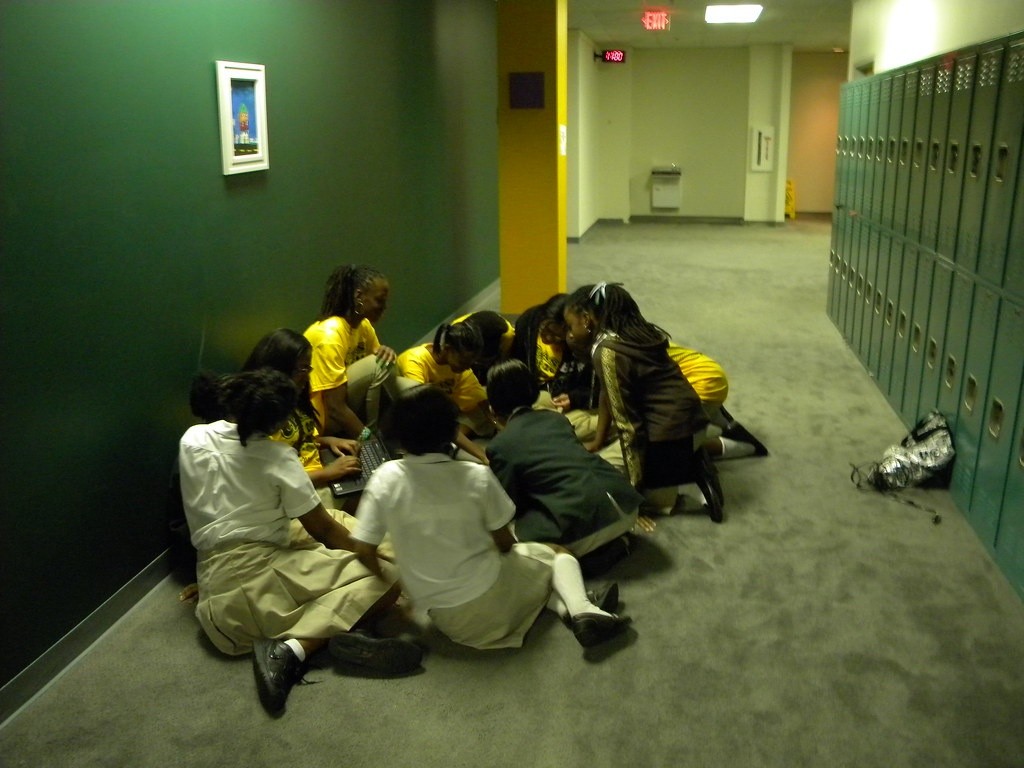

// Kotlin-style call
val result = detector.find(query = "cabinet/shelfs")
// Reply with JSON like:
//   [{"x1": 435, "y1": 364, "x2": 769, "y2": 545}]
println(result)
[{"x1": 825, "y1": 30, "x2": 1023, "y2": 601}]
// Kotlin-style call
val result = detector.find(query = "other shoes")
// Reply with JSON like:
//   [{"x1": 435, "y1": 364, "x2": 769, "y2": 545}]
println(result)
[
  {"x1": 711, "y1": 405, "x2": 734, "y2": 431},
  {"x1": 570, "y1": 612, "x2": 632, "y2": 647},
  {"x1": 621, "y1": 532, "x2": 640, "y2": 554},
  {"x1": 691, "y1": 460, "x2": 723, "y2": 524},
  {"x1": 563, "y1": 582, "x2": 618, "y2": 631},
  {"x1": 693, "y1": 446, "x2": 725, "y2": 507},
  {"x1": 721, "y1": 421, "x2": 768, "y2": 455}
]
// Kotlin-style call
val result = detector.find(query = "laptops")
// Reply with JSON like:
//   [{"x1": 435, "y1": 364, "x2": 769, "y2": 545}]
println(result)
[{"x1": 318, "y1": 382, "x2": 396, "y2": 498}]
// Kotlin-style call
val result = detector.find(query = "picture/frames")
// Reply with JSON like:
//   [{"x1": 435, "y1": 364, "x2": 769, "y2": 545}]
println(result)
[{"x1": 214, "y1": 60, "x2": 269, "y2": 176}]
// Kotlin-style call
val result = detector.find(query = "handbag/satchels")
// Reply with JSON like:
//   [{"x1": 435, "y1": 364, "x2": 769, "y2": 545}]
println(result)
[{"x1": 850, "y1": 408, "x2": 956, "y2": 526}]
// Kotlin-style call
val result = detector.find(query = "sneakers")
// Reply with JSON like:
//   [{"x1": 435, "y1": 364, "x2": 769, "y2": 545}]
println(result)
[
  {"x1": 251, "y1": 638, "x2": 319, "y2": 717},
  {"x1": 328, "y1": 633, "x2": 424, "y2": 679}
]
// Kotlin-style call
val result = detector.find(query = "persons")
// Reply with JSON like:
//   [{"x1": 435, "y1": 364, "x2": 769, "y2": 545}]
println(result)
[{"x1": 179, "y1": 261, "x2": 768, "y2": 715}]
